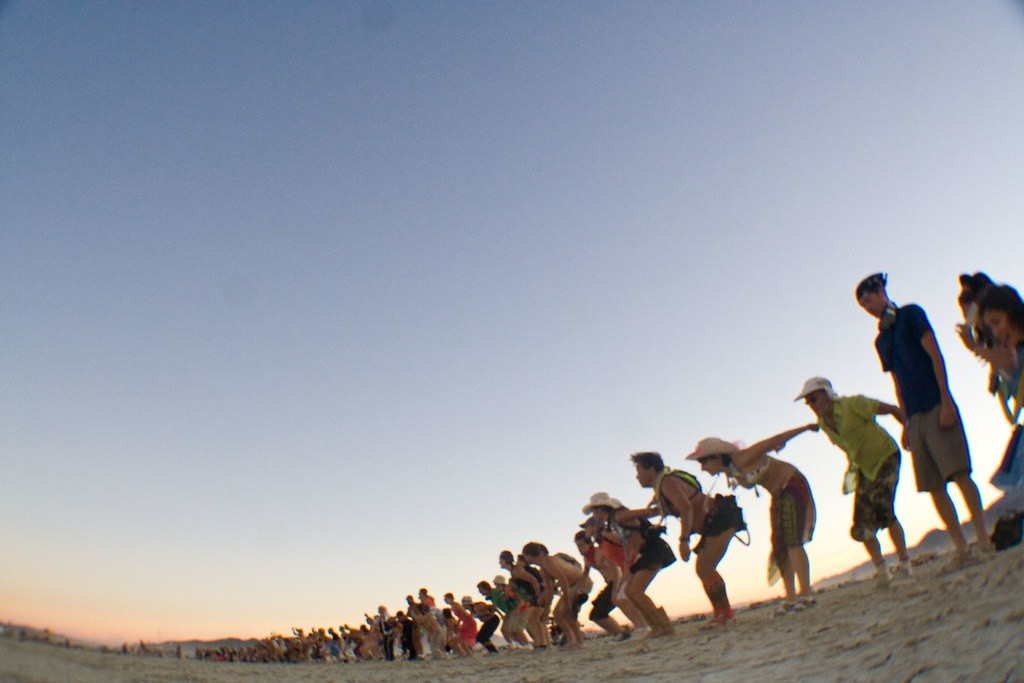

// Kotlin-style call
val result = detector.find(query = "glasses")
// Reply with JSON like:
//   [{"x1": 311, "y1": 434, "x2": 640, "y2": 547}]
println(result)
[{"x1": 805, "y1": 391, "x2": 823, "y2": 404}]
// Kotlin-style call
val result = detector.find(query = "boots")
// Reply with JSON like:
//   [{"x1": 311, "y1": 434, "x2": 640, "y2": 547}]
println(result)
[{"x1": 641, "y1": 607, "x2": 674, "y2": 639}]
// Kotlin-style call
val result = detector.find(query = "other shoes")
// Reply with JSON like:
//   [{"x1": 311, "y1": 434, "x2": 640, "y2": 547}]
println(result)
[
  {"x1": 773, "y1": 597, "x2": 816, "y2": 617},
  {"x1": 702, "y1": 611, "x2": 737, "y2": 631},
  {"x1": 888, "y1": 566, "x2": 915, "y2": 587},
  {"x1": 938, "y1": 549, "x2": 972, "y2": 574},
  {"x1": 871, "y1": 573, "x2": 891, "y2": 590},
  {"x1": 962, "y1": 546, "x2": 996, "y2": 567},
  {"x1": 612, "y1": 624, "x2": 631, "y2": 641}
]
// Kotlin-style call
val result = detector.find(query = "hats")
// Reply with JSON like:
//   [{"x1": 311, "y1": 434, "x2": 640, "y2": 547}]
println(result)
[
  {"x1": 462, "y1": 596, "x2": 473, "y2": 604},
  {"x1": 493, "y1": 575, "x2": 506, "y2": 585},
  {"x1": 583, "y1": 493, "x2": 622, "y2": 515},
  {"x1": 579, "y1": 517, "x2": 605, "y2": 529},
  {"x1": 795, "y1": 377, "x2": 832, "y2": 400},
  {"x1": 685, "y1": 438, "x2": 737, "y2": 458}
]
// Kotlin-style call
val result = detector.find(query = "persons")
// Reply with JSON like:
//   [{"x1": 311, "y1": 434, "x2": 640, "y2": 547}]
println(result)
[
  {"x1": 583, "y1": 492, "x2": 676, "y2": 640},
  {"x1": 477, "y1": 517, "x2": 641, "y2": 652},
  {"x1": 177, "y1": 643, "x2": 183, "y2": 658},
  {"x1": 854, "y1": 271, "x2": 1024, "y2": 569},
  {"x1": 794, "y1": 377, "x2": 903, "y2": 584},
  {"x1": 632, "y1": 452, "x2": 737, "y2": 627},
  {"x1": 686, "y1": 424, "x2": 819, "y2": 617},
  {"x1": 195, "y1": 588, "x2": 499, "y2": 663}
]
